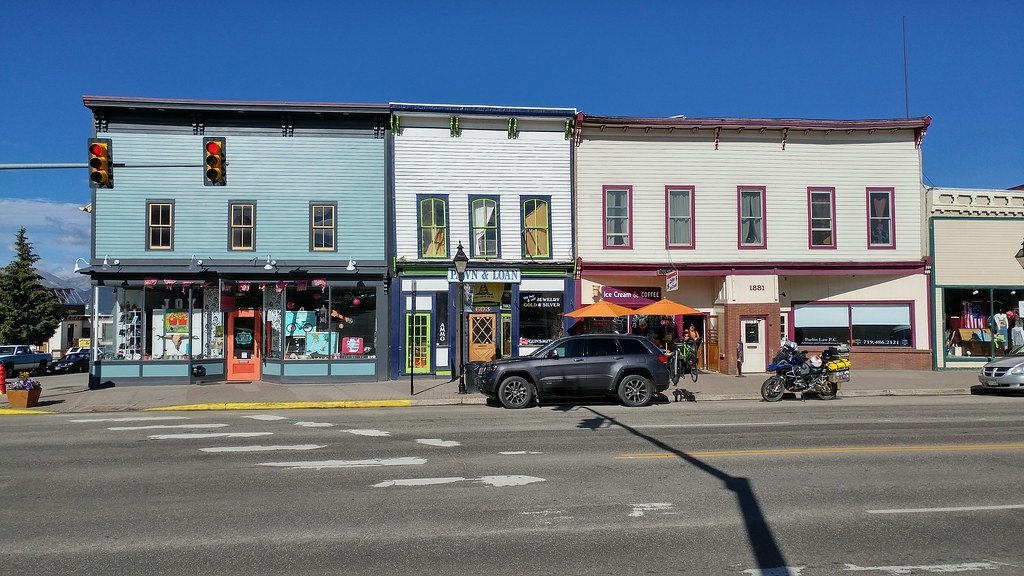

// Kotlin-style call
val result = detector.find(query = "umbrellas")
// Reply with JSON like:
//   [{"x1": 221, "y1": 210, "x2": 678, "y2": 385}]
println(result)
[
  {"x1": 564, "y1": 299, "x2": 636, "y2": 333},
  {"x1": 632, "y1": 297, "x2": 702, "y2": 354}
]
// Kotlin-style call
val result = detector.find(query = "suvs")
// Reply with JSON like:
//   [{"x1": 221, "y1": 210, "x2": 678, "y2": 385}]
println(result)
[{"x1": 476, "y1": 334, "x2": 670, "y2": 409}]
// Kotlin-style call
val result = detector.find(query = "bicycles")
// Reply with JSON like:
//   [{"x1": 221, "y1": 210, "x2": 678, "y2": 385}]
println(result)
[{"x1": 678, "y1": 338, "x2": 698, "y2": 382}]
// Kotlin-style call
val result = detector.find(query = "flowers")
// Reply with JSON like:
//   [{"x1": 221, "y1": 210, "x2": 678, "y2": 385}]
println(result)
[{"x1": 8, "y1": 370, "x2": 41, "y2": 390}]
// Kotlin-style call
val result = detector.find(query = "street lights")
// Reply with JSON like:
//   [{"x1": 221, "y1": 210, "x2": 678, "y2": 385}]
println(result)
[{"x1": 453, "y1": 240, "x2": 469, "y2": 394}]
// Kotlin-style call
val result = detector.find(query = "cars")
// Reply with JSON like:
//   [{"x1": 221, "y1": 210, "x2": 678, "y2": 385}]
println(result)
[
  {"x1": 52, "y1": 347, "x2": 107, "y2": 374},
  {"x1": 978, "y1": 344, "x2": 1024, "y2": 390}
]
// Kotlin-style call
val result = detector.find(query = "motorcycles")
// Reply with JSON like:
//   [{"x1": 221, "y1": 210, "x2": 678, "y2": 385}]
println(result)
[{"x1": 761, "y1": 340, "x2": 851, "y2": 402}]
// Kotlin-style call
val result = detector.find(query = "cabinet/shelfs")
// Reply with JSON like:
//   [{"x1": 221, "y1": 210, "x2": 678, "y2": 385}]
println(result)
[{"x1": 119, "y1": 311, "x2": 141, "y2": 359}]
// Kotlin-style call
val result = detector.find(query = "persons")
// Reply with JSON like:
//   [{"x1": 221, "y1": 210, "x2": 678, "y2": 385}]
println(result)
[{"x1": 685, "y1": 323, "x2": 700, "y2": 338}]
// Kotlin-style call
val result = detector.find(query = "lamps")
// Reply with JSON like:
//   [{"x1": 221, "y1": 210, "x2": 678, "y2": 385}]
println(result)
[
  {"x1": 346, "y1": 255, "x2": 357, "y2": 271},
  {"x1": 190, "y1": 254, "x2": 203, "y2": 270},
  {"x1": 121, "y1": 280, "x2": 129, "y2": 287},
  {"x1": 264, "y1": 254, "x2": 277, "y2": 270},
  {"x1": 73, "y1": 258, "x2": 90, "y2": 273},
  {"x1": 103, "y1": 254, "x2": 120, "y2": 268}
]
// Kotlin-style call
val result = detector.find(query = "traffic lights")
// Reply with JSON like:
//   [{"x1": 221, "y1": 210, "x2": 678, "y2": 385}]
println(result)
[
  {"x1": 88, "y1": 138, "x2": 114, "y2": 189},
  {"x1": 203, "y1": 137, "x2": 227, "y2": 186}
]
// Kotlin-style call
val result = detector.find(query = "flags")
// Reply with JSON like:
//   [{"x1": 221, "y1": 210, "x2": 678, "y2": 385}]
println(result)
[{"x1": 964, "y1": 303, "x2": 984, "y2": 328}]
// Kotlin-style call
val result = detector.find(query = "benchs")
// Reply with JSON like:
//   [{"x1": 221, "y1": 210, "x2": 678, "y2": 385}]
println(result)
[{"x1": 958, "y1": 328, "x2": 1006, "y2": 357}]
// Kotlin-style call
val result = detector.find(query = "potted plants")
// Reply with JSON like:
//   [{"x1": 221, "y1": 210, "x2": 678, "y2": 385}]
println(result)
[{"x1": 119, "y1": 301, "x2": 137, "y2": 311}]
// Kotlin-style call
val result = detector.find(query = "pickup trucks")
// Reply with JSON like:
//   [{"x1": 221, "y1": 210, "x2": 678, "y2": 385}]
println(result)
[{"x1": 0, "y1": 345, "x2": 52, "y2": 378}]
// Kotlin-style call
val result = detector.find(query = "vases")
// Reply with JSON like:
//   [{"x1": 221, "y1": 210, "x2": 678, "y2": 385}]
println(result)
[{"x1": 6, "y1": 388, "x2": 42, "y2": 408}]
